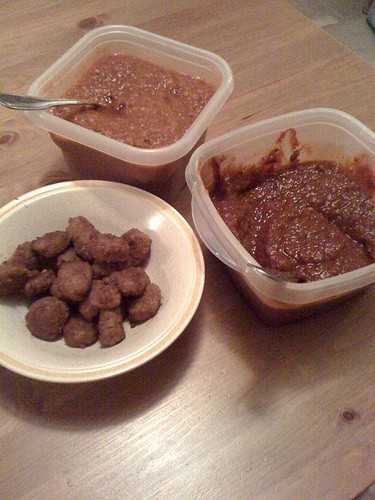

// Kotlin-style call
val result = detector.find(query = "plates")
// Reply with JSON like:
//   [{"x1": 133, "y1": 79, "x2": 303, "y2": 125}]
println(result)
[{"x1": 0, "y1": 179, "x2": 205, "y2": 383}]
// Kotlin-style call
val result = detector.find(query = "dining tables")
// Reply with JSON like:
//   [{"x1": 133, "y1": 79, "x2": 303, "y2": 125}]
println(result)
[{"x1": 0, "y1": 0, "x2": 375, "y2": 500}]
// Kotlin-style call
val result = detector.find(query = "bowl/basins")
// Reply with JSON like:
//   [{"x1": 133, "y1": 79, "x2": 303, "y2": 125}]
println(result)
[
  {"x1": 24, "y1": 23, "x2": 235, "y2": 203},
  {"x1": 185, "y1": 106, "x2": 375, "y2": 327}
]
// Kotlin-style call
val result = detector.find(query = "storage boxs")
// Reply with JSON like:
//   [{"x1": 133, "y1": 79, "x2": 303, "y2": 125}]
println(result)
[
  {"x1": 25, "y1": 24, "x2": 235, "y2": 204},
  {"x1": 185, "y1": 107, "x2": 375, "y2": 326}
]
